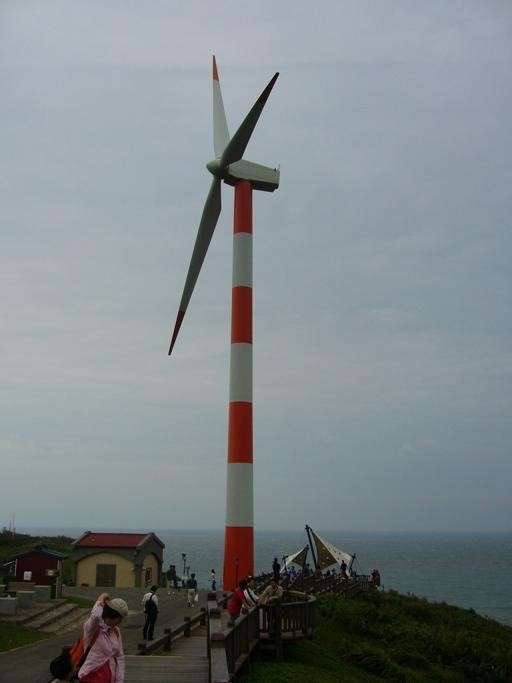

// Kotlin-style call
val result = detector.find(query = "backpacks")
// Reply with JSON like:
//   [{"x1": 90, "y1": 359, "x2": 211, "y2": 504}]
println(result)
[
  {"x1": 69, "y1": 627, "x2": 119, "y2": 676},
  {"x1": 144, "y1": 593, "x2": 159, "y2": 621}
]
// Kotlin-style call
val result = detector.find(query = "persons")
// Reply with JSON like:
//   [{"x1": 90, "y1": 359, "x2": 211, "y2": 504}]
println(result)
[
  {"x1": 141, "y1": 585, "x2": 158, "y2": 640},
  {"x1": 228, "y1": 557, "x2": 349, "y2": 621},
  {"x1": 167, "y1": 565, "x2": 177, "y2": 595},
  {"x1": 210, "y1": 568, "x2": 217, "y2": 592},
  {"x1": 187, "y1": 573, "x2": 197, "y2": 608},
  {"x1": 79, "y1": 593, "x2": 128, "y2": 682}
]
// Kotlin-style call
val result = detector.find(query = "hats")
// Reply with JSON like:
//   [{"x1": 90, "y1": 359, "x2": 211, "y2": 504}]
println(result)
[{"x1": 104, "y1": 598, "x2": 128, "y2": 617}]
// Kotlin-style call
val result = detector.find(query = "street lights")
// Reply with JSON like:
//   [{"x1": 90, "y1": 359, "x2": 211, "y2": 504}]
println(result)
[
  {"x1": 231, "y1": 554, "x2": 242, "y2": 590},
  {"x1": 179, "y1": 551, "x2": 187, "y2": 576},
  {"x1": 186, "y1": 564, "x2": 191, "y2": 585}
]
